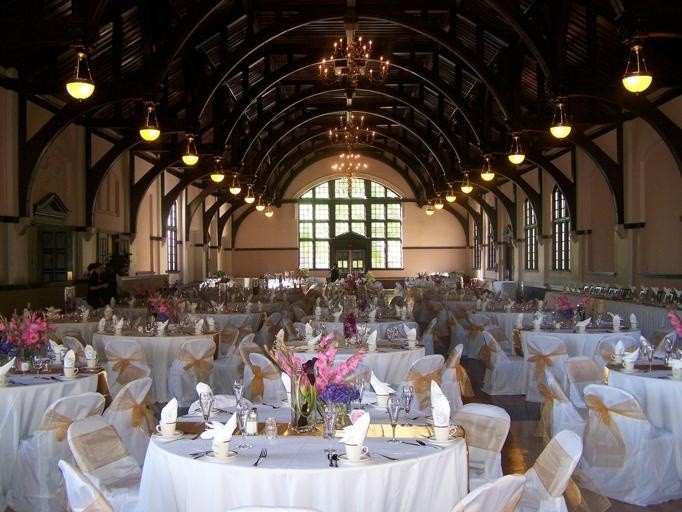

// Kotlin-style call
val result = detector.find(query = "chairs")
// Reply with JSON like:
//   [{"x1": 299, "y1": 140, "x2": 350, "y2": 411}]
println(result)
[{"x1": 0, "y1": 276, "x2": 682, "y2": 509}]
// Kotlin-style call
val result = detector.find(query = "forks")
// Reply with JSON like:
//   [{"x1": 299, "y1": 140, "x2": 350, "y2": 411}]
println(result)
[{"x1": 253, "y1": 448, "x2": 267, "y2": 466}]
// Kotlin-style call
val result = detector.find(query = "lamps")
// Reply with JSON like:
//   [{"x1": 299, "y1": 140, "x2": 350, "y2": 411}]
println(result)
[
  {"x1": 420, "y1": 3, "x2": 574, "y2": 219},
  {"x1": 64, "y1": 0, "x2": 97, "y2": 106},
  {"x1": 131, "y1": 49, "x2": 275, "y2": 222},
  {"x1": 316, "y1": 0, "x2": 389, "y2": 198},
  {"x1": 620, "y1": 0, "x2": 653, "y2": 95}
]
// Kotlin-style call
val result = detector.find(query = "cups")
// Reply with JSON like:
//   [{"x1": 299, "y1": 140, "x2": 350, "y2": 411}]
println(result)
[
  {"x1": 433, "y1": 425, "x2": 456, "y2": 441},
  {"x1": 86, "y1": 359, "x2": 97, "y2": 368},
  {"x1": 63, "y1": 367, "x2": 78, "y2": 376},
  {"x1": 344, "y1": 444, "x2": 368, "y2": 460},
  {"x1": 264, "y1": 418, "x2": 277, "y2": 440},
  {"x1": 212, "y1": 440, "x2": 230, "y2": 456},
  {"x1": 609, "y1": 353, "x2": 682, "y2": 379},
  {"x1": 377, "y1": 395, "x2": 389, "y2": 406},
  {"x1": 408, "y1": 340, "x2": 416, "y2": 347},
  {"x1": 156, "y1": 420, "x2": 176, "y2": 436}
]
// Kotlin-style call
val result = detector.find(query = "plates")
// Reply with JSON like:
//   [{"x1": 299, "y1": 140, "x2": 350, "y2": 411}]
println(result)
[
  {"x1": 152, "y1": 429, "x2": 183, "y2": 438},
  {"x1": 337, "y1": 453, "x2": 373, "y2": 463},
  {"x1": 204, "y1": 450, "x2": 238, "y2": 460}
]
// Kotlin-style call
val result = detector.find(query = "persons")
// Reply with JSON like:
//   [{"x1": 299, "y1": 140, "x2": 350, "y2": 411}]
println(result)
[
  {"x1": 331, "y1": 264, "x2": 340, "y2": 281},
  {"x1": 86, "y1": 262, "x2": 107, "y2": 309}
]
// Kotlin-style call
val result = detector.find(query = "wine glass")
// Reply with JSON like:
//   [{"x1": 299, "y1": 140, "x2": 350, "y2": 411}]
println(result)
[
  {"x1": 199, "y1": 391, "x2": 212, "y2": 421},
  {"x1": 31, "y1": 355, "x2": 44, "y2": 378},
  {"x1": 386, "y1": 398, "x2": 400, "y2": 443},
  {"x1": 355, "y1": 378, "x2": 365, "y2": 410},
  {"x1": 402, "y1": 385, "x2": 414, "y2": 417},
  {"x1": 323, "y1": 404, "x2": 336, "y2": 459}
]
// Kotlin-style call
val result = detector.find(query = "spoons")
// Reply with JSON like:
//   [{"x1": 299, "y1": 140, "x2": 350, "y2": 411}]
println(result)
[{"x1": 332, "y1": 454, "x2": 338, "y2": 467}]
